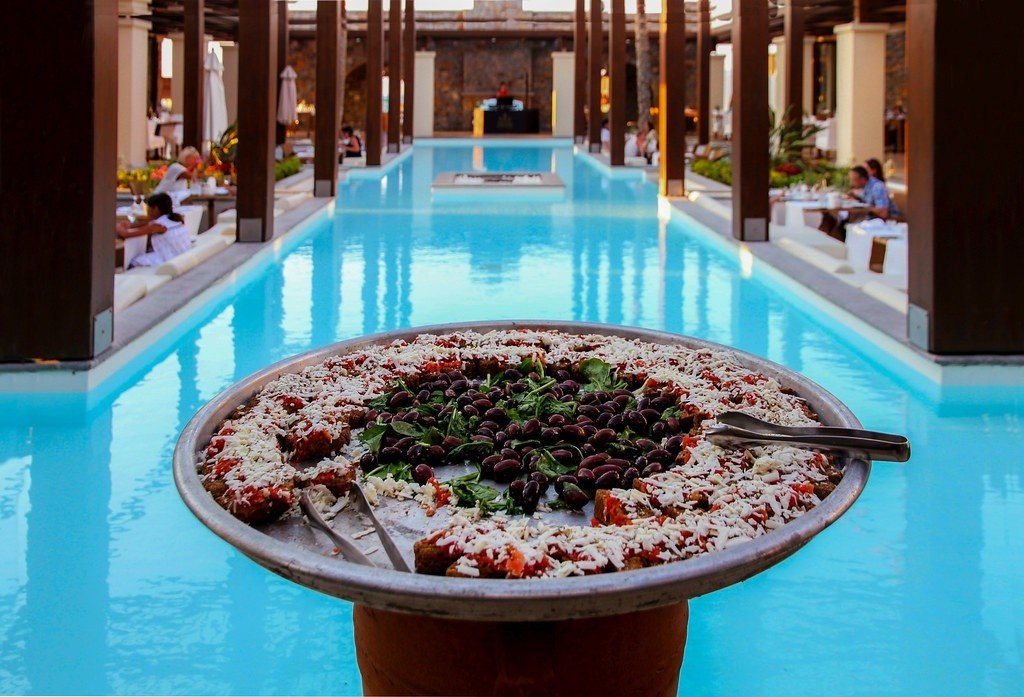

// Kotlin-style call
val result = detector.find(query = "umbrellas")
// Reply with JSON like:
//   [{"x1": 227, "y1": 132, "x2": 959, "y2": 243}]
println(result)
[{"x1": 277, "y1": 65, "x2": 298, "y2": 126}]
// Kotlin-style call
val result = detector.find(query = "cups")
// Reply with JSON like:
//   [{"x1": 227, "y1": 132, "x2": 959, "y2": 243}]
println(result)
[{"x1": 224, "y1": 176, "x2": 231, "y2": 185}]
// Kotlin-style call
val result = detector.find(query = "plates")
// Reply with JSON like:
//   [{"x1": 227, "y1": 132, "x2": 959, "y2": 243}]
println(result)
[{"x1": 173, "y1": 321, "x2": 870, "y2": 621}]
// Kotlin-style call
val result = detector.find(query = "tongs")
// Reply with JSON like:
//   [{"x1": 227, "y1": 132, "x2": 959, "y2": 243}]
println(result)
[
  {"x1": 703, "y1": 411, "x2": 910, "y2": 462},
  {"x1": 299, "y1": 481, "x2": 414, "y2": 575}
]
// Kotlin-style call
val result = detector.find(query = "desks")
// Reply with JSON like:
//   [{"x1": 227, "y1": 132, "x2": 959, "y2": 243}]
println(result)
[
  {"x1": 847, "y1": 222, "x2": 908, "y2": 290},
  {"x1": 774, "y1": 192, "x2": 870, "y2": 227},
  {"x1": 184, "y1": 192, "x2": 237, "y2": 230}
]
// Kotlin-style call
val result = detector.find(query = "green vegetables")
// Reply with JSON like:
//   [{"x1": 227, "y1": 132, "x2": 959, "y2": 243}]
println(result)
[{"x1": 360, "y1": 358, "x2": 682, "y2": 509}]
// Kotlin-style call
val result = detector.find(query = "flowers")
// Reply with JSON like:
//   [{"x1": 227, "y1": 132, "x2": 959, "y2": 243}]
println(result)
[
  {"x1": 115, "y1": 120, "x2": 239, "y2": 193},
  {"x1": 768, "y1": 103, "x2": 852, "y2": 189}
]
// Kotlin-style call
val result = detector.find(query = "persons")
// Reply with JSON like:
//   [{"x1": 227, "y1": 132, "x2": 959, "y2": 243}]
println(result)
[
  {"x1": 816, "y1": 160, "x2": 903, "y2": 243},
  {"x1": 116, "y1": 192, "x2": 193, "y2": 271},
  {"x1": 150, "y1": 146, "x2": 210, "y2": 208},
  {"x1": 601, "y1": 117, "x2": 659, "y2": 158},
  {"x1": 497, "y1": 81, "x2": 510, "y2": 99},
  {"x1": 338, "y1": 126, "x2": 362, "y2": 163},
  {"x1": 886, "y1": 105, "x2": 904, "y2": 152}
]
